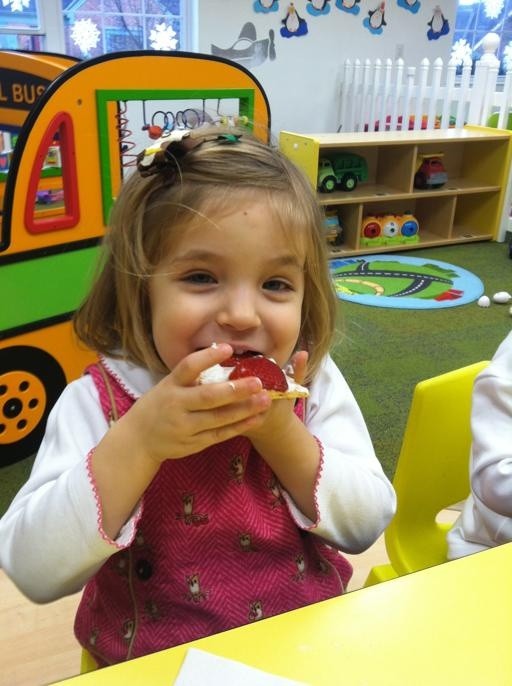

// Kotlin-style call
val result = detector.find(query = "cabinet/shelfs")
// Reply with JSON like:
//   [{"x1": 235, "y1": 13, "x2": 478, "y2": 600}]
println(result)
[{"x1": 277, "y1": 125, "x2": 512, "y2": 259}]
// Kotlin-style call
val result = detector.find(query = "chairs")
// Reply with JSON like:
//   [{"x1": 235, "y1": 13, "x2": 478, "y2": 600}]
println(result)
[{"x1": 352, "y1": 358, "x2": 503, "y2": 590}]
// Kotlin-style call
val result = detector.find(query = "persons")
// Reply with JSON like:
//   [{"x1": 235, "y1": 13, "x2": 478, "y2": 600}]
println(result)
[
  {"x1": 448, "y1": 328, "x2": 512, "y2": 561},
  {"x1": 0, "y1": 126, "x2": 396, "y2": 672}
]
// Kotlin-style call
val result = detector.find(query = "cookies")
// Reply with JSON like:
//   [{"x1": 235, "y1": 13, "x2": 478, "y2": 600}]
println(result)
[{"x1": 198, "y1": 351, "x2": 310, "y2": 401}]
